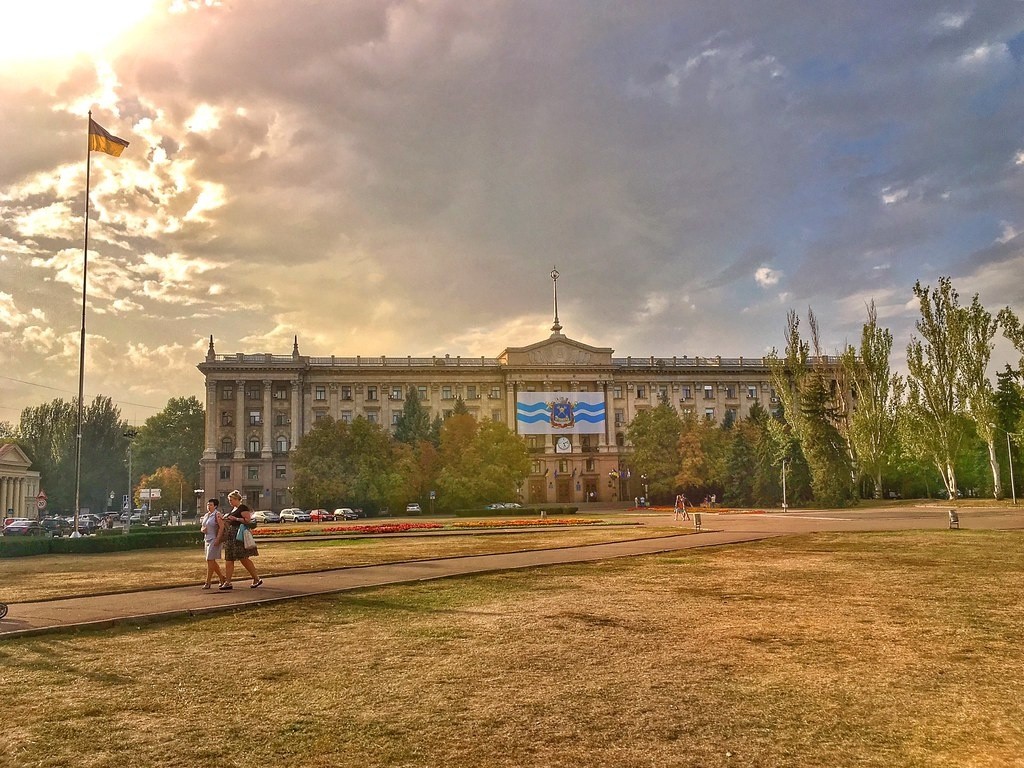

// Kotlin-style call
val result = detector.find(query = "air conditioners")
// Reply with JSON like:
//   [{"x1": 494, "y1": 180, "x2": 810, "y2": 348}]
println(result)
[
  {"x1": 746, "y1": 394, "x2": 752, "y2": 398},
  {"x1": 253, "y1": 475, "x2": 257, "y2": 479},
  {"x1": 615, "y1": 422, "x2": 622, "y2": 427},
  {"x1": 628, "y1": 384, "x2": 634, "y2": 390},
  {"x1": 388, "y1": 395, "x2": 394, "y2": 399},
  {"x1": 451, "y1": 394, "x2": 457, "y2": 399},
  {"x1": 245, "y1": 392, "x2": 251, "y2": 396},
  {"x1": 281, "y1": 474, "x2": 286, "y2": 478},
  {"x1": 680, "y1": 397, "x2": 686, "y2": 402},
  {"x1": 723, "y1": 387, "x2": 728, "y2": 391},
  {"x1": 771, "y1": 398, "x2": 777, "y2": 403},
  {"x1": 476, "y1": 394, "x2": 481, "y2": 399},
  {"x1": 273, "y1": 393, "x2": 278, "y2": 398},
  {"x1": 286, "y1": 419, "x2": 291, "y2": 423}
]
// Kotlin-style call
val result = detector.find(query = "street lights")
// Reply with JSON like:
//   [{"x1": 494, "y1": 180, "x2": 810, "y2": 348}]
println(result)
[
  {"x1": 123, "y1": 429, "x2": 138, "y2": 516},
  {"x1": 988, "y1": 422, "x2": 1016, "y2": 504}
]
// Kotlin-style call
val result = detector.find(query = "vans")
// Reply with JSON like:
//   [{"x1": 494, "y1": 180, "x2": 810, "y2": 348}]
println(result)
[{"x1": 104, "y1": 511, "x2": 120, "y2": 521}]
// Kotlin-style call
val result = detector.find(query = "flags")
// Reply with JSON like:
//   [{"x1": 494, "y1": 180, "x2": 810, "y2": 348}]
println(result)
[
  {"x1": 544, "y1": 468, "x2": 550, "y2": 477},
  {"x1": 554, "y1": 469, "x2": 557, "y2": 478},
  {"x1": 89, "y1": 118, "x2": 130, "y2": 157},
  {"x1": 611, "y1": 469, "x2": 625, "y2": 478}
]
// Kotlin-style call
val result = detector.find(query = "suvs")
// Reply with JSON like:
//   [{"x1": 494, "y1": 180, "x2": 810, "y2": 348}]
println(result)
[
  {"x1": 309, "y1": 509, "x2": 334, "y2": 522},
  {"x1": 280, "y1": 508, "x2": 310, "y2": 523},
  {"x1": 406, "y1": 502, "x2": 422, "y2": 515},
  {"x1": 40, "y1": 518, "x2": 73, "y2": 537},
  {"x1": 334, "y1": 508, "x2": 358, "y2": 520}
]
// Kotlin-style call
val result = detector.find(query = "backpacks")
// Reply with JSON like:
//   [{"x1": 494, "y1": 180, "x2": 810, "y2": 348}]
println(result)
[{"x1": 678, "y1": 500, "x2": 683, "y2": 510}]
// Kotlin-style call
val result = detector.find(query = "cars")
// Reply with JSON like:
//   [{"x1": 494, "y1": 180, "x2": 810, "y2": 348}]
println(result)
[
  {"x1": 4, "y1": 513, "x2": 113, "y2": 530},
  {"x1": 489, "y1": 503, "x2": 521, "y2": 509},
  {"x1": 119, "y1": 513, "x2": 129, "y2": 523},
  {"x1": 2, "y1": 520, "x2": 48, "y2": 537},
  {"x1": 130, "y1": 514, "x2": 146, "y2": 524},
  {"x1": 69, "y1": 520, "x2": 98, "y2": 536},
  {"x1": 378, "y1": 507, "x2": 392, "y2": 516},
  {"x1": 254, "y1": 510, "x2": 280, "y2": 524},
  {"x1": 159, "y1": 510, "x2": 169, "y2": 517},
  {"x1": 148, "y1": 516, "x2": 168, "y2": 527}
]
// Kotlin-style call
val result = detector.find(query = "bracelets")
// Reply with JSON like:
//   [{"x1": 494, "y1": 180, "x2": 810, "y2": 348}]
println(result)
[{"x1": 237, "y1": 518, "x2": 238, "y2": 521}]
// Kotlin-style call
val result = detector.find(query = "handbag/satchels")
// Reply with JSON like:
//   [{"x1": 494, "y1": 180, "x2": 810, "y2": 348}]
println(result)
[
  {"x1": 243, "y1": 518, "x2": 257, "y2": 549},
  {"x1": 236, "y1": 517, "x2": 247, "y2": 542},
  {"x1": 213, "y1": 512, "x2": 230, "y2": 542}
]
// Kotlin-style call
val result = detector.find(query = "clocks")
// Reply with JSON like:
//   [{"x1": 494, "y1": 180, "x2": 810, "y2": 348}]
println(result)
[{"x1": 558, "y1": 437, "x2": 570, "y2": 450}]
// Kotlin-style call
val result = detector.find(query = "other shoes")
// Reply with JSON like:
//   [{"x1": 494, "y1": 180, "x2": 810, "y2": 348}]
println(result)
[
  {"x1": 201, "y1": 583, "x2": 211, "y2": 589},
  {"x1": 218, "y1": 576, "x2": 227, "y2": 587}
]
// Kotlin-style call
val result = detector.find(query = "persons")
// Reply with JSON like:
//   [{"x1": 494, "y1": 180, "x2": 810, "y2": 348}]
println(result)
[
  {"x1": 673, "y1": 494, "x2": 693, "y2": 521},
  {"x1": 897, "y1": 489, "x2": 901, "y2": 500},
  {"x1": 635, "y1": 496, "x2": 638, "y2": 508},
  {"x1": 218, "y1": 490, "x2": 263, "y2": 590},
  {"x1": 706, "y1": 493, "x2": 716, "y2": 508},
  {"x1": 201, "y1": 498, "x2": 226, "y2": 589},
  {"x1": 592, "y1": 491, "x2": 597, "y2": 502},
  {"x1": 640, "y1": 496, "x2": 645, "y2": 507},
  {"x1": 101, "y1": 517, "x2": 106, "y2": 528},
  {"x1": 106, "y1": 515, "x2": 113, "y2": 529}
]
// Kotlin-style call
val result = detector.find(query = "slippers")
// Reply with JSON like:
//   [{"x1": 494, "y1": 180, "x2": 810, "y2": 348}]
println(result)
[
  {"x1": 250, "y1": 578, "x2": 263, "y2": 588},
  {"x1": 219, "y1": 582, "x2": 233, "y2": 590}
]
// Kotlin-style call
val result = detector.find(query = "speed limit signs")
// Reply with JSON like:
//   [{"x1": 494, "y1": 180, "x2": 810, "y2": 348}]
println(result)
[{"x1": 37, "y1": 500, "x2": 46, "y2": 509}]
